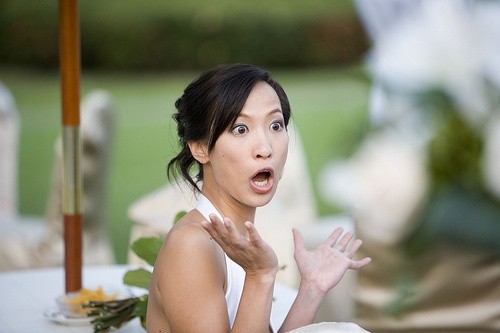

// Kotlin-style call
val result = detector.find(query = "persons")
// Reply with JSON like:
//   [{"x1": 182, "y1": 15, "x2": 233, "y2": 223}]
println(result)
[{"x1": 145, "y1": 62, "x2": 373, "y2": 333}]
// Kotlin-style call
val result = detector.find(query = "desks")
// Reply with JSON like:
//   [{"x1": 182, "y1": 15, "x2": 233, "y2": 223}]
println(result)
[{"x1": 0, "y1": 266, "x2": 300, "y2": 333}]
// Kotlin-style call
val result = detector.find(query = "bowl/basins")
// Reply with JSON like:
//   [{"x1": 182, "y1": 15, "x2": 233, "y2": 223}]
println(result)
[{"x1": 55, "y1": 293, "x2": 120, "y2": 318}]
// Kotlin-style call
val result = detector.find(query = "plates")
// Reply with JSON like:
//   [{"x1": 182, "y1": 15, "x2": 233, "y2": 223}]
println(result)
[{"x1": 43, "y1": 309, "x2": 100, "y2": 327}]
